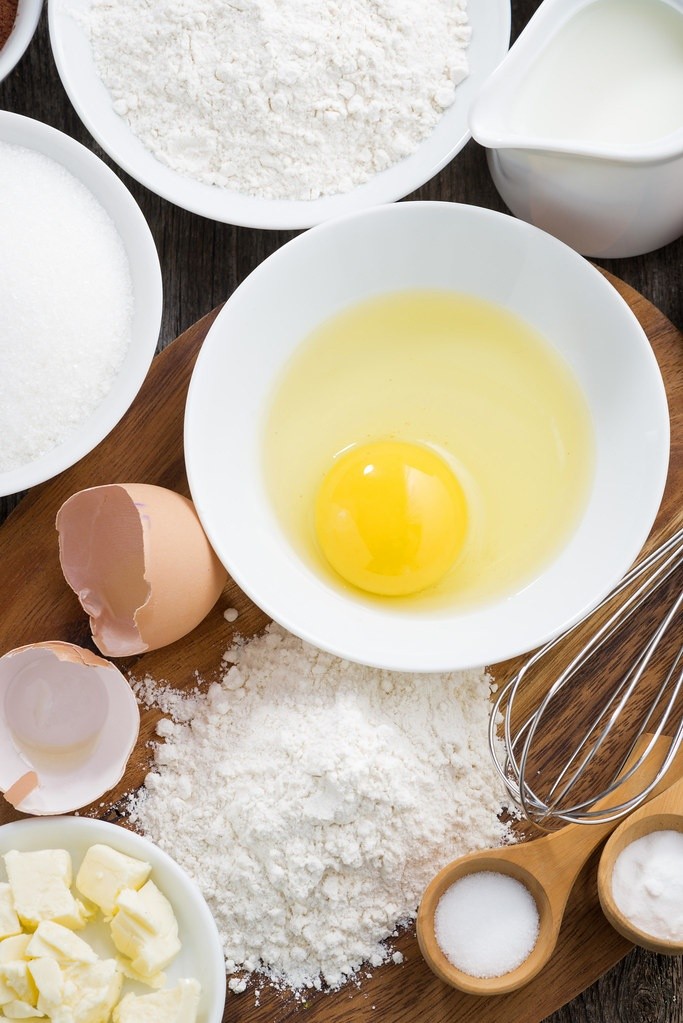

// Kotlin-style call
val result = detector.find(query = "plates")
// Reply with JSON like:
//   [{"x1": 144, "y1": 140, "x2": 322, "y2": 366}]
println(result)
[
  {"x1": 47, "y1": 0, "x2": 512, "y2": 230},
  {"x1": 183, "y1": 201, "x2": 672, "y2": 674},
  {"x1": 0, "y1": 816, "x2": 226, "y2": 1023},
  {"x1": 0, "y1": 109, "x2": 163, "y2": 498}
]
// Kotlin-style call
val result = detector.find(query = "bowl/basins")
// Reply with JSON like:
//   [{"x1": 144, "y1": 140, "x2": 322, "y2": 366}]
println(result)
[{"x1": 0, "y1": 0, "x2": 44, "y2": 83}]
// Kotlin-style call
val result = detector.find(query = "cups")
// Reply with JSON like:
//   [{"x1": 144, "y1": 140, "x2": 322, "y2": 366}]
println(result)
[{"x1": 469, "y1": 0, "x2": 683, "y2": 259}]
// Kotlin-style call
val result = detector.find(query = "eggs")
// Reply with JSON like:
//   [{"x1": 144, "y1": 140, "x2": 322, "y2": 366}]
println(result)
[{"x1": 258, "y1": 284, "x2": 596, "y2": 617}]
